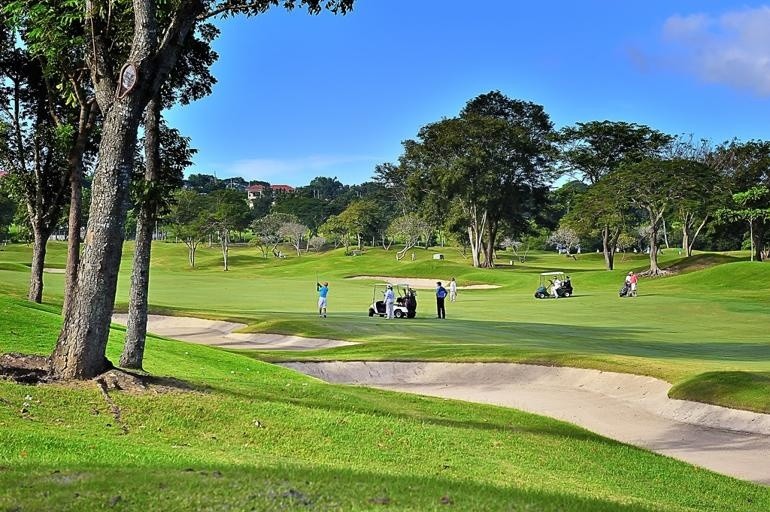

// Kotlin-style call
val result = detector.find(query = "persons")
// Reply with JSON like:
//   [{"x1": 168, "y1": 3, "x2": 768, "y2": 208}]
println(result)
[
  {"x1": 316, "y1": 281, "x2": 329, "y2": 317},
  {"x1": 382, "y1": 285, "x2": 395, "y2": 320},
  {"x1": 450, "y1": 277, "x2": 457, "y2": 303},
  {"x1": 626, "y1": 272, "x2": 631, "y2": 296},
  {"x1": 436, "y1": 282, "x2": 447, "y2": 319},
  {"x1": 629, "y1": 270, "x2": 637, "y2": 296},
  {"x1": 551, "y1": 276, "x2": 561, "y2": 300},
  {"x1": 564, "y1": 276, "x2": 571, "y2": 288}
]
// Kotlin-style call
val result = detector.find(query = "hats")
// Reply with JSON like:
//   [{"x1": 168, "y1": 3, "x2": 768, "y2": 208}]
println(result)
[
  {"x1": 323, "y1": 281, "x2": 328, "y2": 286},
  {"x1": 386, "y1": 284, "x2": 392, "y2": 288}
]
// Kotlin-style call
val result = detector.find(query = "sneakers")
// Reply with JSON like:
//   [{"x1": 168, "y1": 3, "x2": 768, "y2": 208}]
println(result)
[{"x1": 319, "y1": 314, "x2": 327, "y2": 318}]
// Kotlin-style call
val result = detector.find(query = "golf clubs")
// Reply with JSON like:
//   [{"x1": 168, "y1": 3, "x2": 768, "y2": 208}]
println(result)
[
  {"x1": 317, "y1": 274, "x2": 318, "y2": 287},
  {"x1": 402, "y1": 288, "x2": 415, "y2": 297}
]
